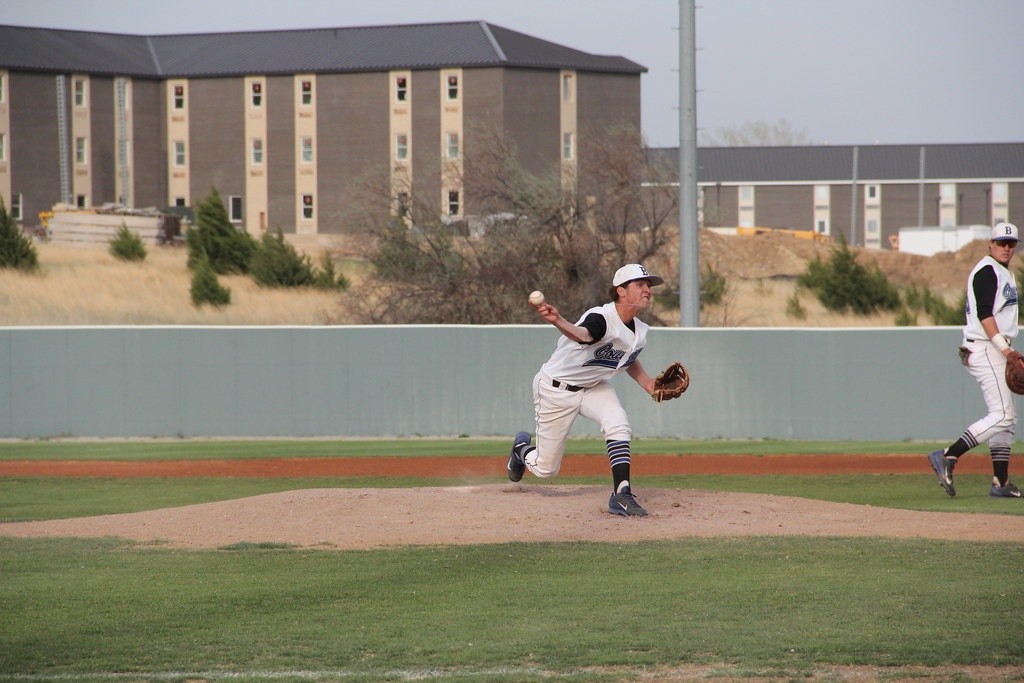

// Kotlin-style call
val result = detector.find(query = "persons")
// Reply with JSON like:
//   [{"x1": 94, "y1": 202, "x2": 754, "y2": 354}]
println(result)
[
  {"x1": 507, "y1": 265, "x2": 689, "y2": 518},
  {"x1": 927, "y1": 221, "x2": 1024, "y2": 498}
]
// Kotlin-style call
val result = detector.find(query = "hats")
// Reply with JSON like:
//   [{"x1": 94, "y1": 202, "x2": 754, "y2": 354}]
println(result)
[
  {"x1": 613, "y1": 263, "x2": 664, "y2": 286},
  {"x1": 991, "y1": 222, "x2": 1022, "y2": 240}
]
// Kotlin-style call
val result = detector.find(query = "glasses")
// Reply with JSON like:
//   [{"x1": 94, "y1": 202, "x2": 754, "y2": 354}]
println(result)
[{"x1": 996, "y1": 239, "x2": 1015, "y2": 247}]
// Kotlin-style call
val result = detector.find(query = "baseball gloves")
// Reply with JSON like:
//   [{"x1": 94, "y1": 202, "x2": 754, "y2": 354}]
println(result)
[
  {"x1": 651, "y1": 361, "x2": 690, "y2": 403},
  {"x1": 1004, "y1": 350, "x2": 1024, "y2": 395}
]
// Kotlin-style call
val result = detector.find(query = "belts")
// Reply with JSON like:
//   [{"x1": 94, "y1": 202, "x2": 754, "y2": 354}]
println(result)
[
  {"x1": 967, "y1": 338, "x2": 974, "y2": 341},
  {"x1": 552, "y1": 379, "x2": 584, "y2": 391}
]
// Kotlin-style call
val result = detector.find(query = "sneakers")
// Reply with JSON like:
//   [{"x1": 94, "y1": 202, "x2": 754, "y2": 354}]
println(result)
[
  {"x1": 927, "y1": 446, "x2": 958, "y2": 496},
  {"x1": 989, "y1": 476, "x2": 1024, "y2": 497},
  {"x1": 607, "y1": 485, "x2": 648, "y2": 516},
  {"x1": 507, "y1": 431, "x2": 532, "y2": 482}
]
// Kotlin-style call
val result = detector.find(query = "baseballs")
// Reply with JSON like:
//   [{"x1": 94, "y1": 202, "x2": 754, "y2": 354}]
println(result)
[{"x1": 529, "y1": 290, "x2": 545, "y2": 305}]
